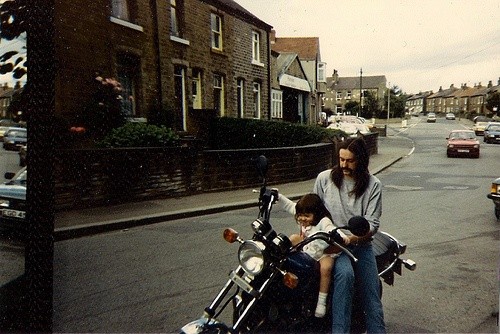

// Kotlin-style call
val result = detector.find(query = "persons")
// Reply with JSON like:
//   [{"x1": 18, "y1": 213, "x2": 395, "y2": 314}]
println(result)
[
  {"x1": 312, "y1": 137, "x2": 387, "y2": 334},
  {"x1": 276, "y1": 193, "x2": 350, "y2": 317}
]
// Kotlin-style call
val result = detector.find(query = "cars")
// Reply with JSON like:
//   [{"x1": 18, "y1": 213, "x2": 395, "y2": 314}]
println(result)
[
  {"x1": 484, "y1": 175, "x2": 500, "y2": 218},
  {"x1": 445, "y1": 112, "x2": 455, "y2": 120},
  {"x1": 2, "y1": 127, "x2": 27, "y2": 151},
  {"x1": 482, "y1": 121, "x2": 500, "y2": 144},
  {"x1": 0, "y1": 163, "x2": 28, "y2": 234},
  {"x1": 18, "y1": 140, "x2": 27, "y2": 166},
  {"x1": 0, "y1": 126, "x2": 10, "y2": 142},
  {"x1": 426, "y1": 112, "x2": 437, "y2": 123},
  {"x1": 473, "y1": 122, "x2": 489, "y2": 136},
  {"x1": 445, "y1": 129, "x2": 481, "y2": 158},
  {"x1": 473, "y1": 116, "x2": 494, "y2": 124}
]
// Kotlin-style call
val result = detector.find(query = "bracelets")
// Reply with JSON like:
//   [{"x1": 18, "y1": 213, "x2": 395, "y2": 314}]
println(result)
[{"x1": 352, "y1": 235, "x2": 359, "y2": 243}]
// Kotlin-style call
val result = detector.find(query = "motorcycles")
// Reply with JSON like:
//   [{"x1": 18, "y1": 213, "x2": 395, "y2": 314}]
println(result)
[{"x1": 175, "y1": 154, "x2": 417, "y2": 334}]
